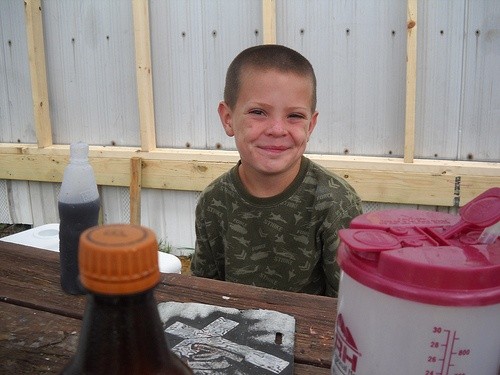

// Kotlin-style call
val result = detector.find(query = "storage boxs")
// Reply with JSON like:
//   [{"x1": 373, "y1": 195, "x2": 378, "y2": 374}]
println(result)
[{"x1": 0, "y1": 223, "x2": 183, "y2": 275}]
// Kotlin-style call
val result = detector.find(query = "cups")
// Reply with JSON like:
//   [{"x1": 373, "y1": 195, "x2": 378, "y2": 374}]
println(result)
[{"x1": 331, "y1": 187, "x2": 500, "y2": 375}]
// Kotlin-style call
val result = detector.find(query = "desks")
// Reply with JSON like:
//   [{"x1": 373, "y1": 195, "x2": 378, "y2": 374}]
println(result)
[{"x1": 0, "y1": 241, "x2": 338, "y2": 375}]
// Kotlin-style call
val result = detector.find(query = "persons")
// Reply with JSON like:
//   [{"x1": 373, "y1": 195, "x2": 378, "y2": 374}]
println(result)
[{"x1": 189, "y1": 44, "x2": 364, "y2": 298}]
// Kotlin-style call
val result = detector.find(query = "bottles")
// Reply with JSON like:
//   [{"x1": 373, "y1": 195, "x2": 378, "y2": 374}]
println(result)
[
  {"x1": 61, "y1": 224, "x2": 193, "y2": 375},
  {"x1": 57, "y1": 144, "x2": 100, "y2": 295}
]
